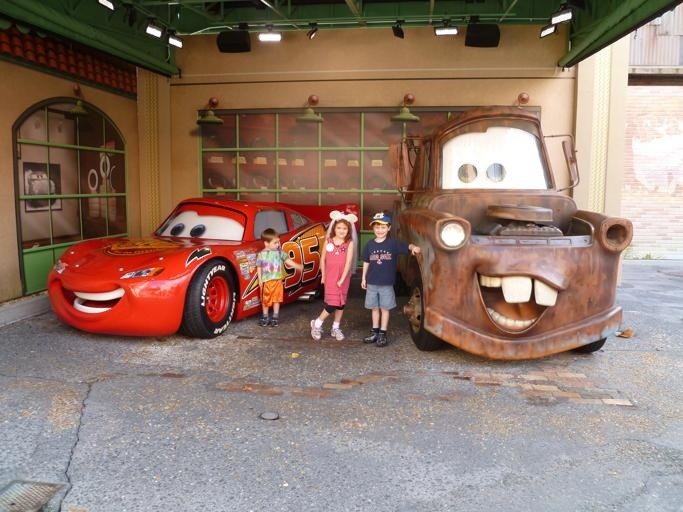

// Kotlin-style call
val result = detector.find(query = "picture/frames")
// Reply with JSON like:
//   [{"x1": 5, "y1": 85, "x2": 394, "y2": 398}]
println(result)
[
  {"x1": 15, "y1": 127, "x2": 21, "y2": 160},
  {"x1": 22, "y1": 161, "x2": 62, "y2": 212}
]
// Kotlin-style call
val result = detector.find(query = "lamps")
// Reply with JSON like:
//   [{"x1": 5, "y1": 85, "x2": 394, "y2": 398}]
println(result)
[
  {"x1": 387, "y1": 92, "x2": 421, "y2": 124},
  {"x1": 517, "y1": 92, "x2": 530, "y2": 110},
  {"x1": 550, "y1": 9, "x2": 573, "y2": 24},
  {"x1": 538, "y1": 23, "x2": 556, "y2": 37},
  {"x1": 167, "y1": 34, "x2": 183, "y2": 48},
  {"x1": 391, "y1": 22, "x2": 404, "y2": 38},
  {"x1": 257, "y1": 31, "x2": 281, "y2": 42},
  {"x1": 433, "y1": 22, "x2": 459, "y2": 36},
  {"x1": 294, "y1": 95, "x2": 325, "y2": 123},
  {"x1": 145, "y1": 21, "x2": 163, "y2": 38},
  {"x1": 99, "y1": 0, "x2": 116, "y2": 13},
  {"x1": 307, "y1": 26, "x2": 318, "y2": 40},
  {"x1": 65, "y1": 83, "x2": 88, "y2": 118},
  {"x1": 195, "y1": 96, "x2": 223, "y2": 127}
]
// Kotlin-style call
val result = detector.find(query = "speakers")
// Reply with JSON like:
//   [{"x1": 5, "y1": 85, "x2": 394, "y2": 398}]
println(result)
[
  {"x1": 215, "y1": 31, "x2": 251, "y2": 53},
  {"x1": 464, "y1": 24, "x2": 500, "y2": 48}
]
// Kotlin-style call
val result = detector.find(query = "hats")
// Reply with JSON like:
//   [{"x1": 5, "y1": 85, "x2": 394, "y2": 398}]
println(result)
[{"x1": 368, "y1": 212, "x2": 393, "y2": 226}]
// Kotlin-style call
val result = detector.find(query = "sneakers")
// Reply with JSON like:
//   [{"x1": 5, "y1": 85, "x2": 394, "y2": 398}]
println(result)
[
  {"x1": 310, "y1": 319, "x2": 324, "y2": 340},
  {"x1": 330, "y1": 326, "x2": 345, "y2": 341},
  {"x1": 363, "y1": 329, "x2": 377, "y2": 343},
  {"x1": 257, "y1": 315, "x2": 270, "y2": 327},
  {"x1": 376, "y1": 332, "x2": 388, "y2": 346},
  {"x1": 271, "y1": 316, "x2": 279, "y2": 328}
]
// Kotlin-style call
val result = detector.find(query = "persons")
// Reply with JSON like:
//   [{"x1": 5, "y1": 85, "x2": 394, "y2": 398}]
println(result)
[
  {"x1": 310, "y1": 210, "x2": 358, "y2": 341},
  {"x1": 255, "y1": 228, "x2": 304, "y2": 327},
  {"x1": 360, "y1": 211, "x2": 421, "y2": 347}
]
